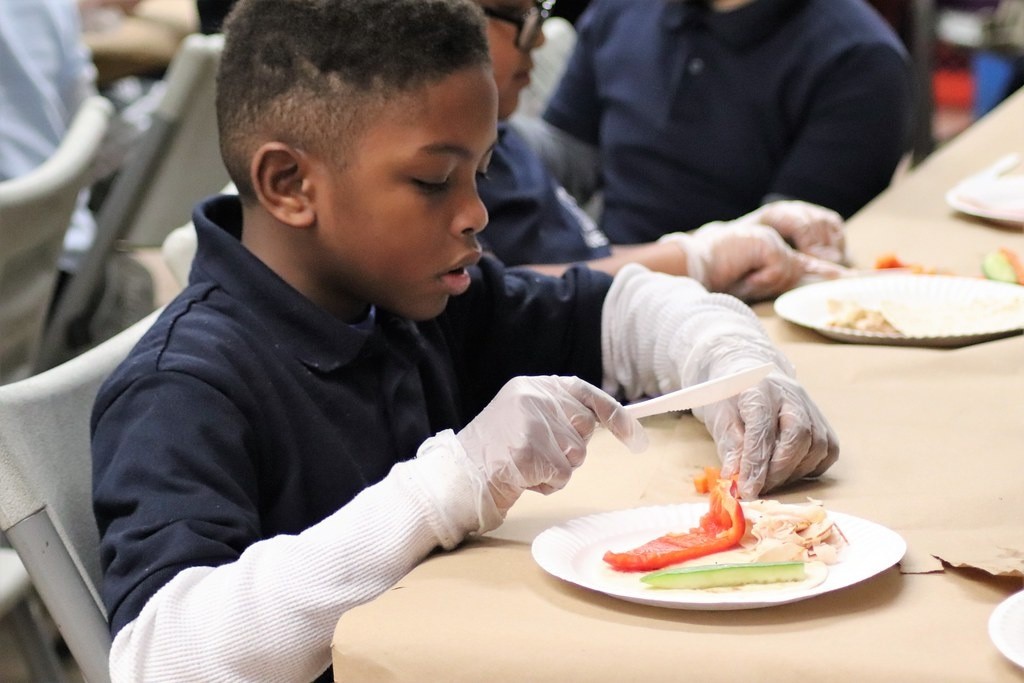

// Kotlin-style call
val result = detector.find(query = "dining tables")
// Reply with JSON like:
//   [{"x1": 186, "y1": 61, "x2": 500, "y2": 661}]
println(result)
[{"x1": 329, "y1": 85, "x2": 1024, "y2": 683}]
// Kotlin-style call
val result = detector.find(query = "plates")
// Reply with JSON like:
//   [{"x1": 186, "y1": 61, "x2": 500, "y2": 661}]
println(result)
[
  {"x1": 948, "y1": 176, "x2": 1024, "y2": 228},
  {"x1": 989, "y1": 589, "x2": 1024, "y2": 669},
  {"x1": 774, "y1": 273, "x2": 1024, "y2": 347},
  {"x1": 531, "y1": 502, "x2": 907, "y2": 610}
]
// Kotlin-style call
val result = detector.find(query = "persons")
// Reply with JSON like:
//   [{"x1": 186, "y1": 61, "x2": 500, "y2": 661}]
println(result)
[
  {"x1": 1, "y1": 0, "x2": 201, "y2": 355},
  {"x1": 510, "y1": 0, "x2": 916, "y2": 247},
  {"x1": 85, "y1": 1, "x2": 841, "y2": 683},
  {"x1": 458, "y1": 0, "x2": 860, "y2": 317}
]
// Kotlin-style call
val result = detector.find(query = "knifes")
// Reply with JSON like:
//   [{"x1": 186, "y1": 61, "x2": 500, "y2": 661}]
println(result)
[{"x1": 623, "y1": 363, "x2": 772, "y2": 418}]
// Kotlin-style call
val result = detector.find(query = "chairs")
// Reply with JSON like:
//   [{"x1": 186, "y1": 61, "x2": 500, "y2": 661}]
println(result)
[
  {"x1": 0, "y1": 302, "x2": 172, "y2": 683},
  {"x1": 0, "y1": 95, "x2": 114, "y2": 683},
  {"x1": 30, "y1": 33, "x2": 228, "y2": 383}
]
